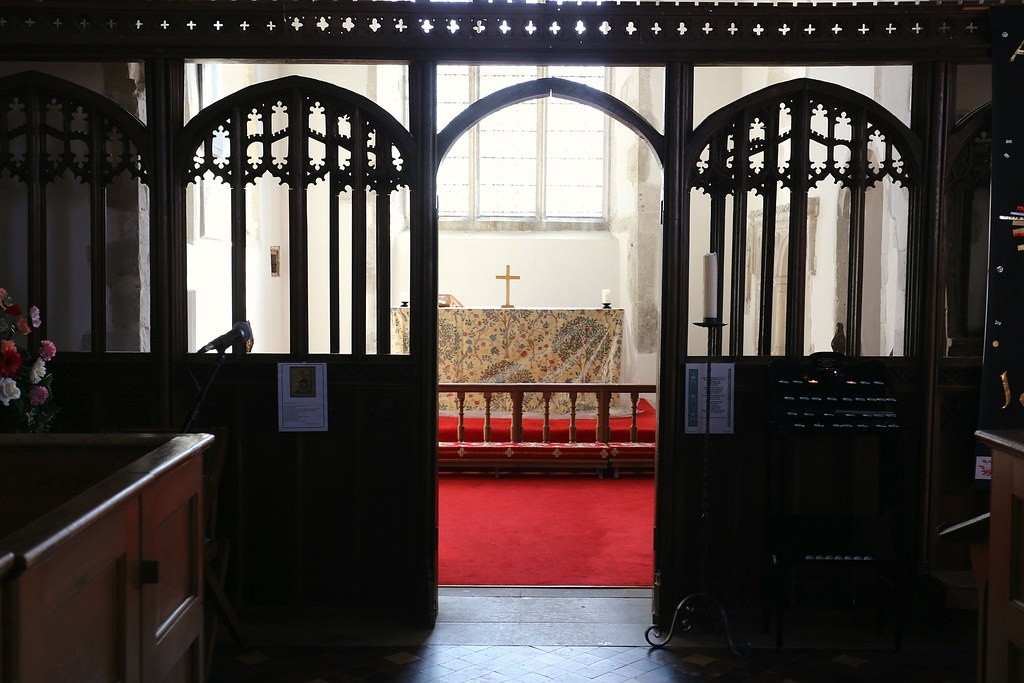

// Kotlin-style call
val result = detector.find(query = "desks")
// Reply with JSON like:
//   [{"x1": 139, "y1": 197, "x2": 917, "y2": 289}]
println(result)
[{"x1": 976, "y1": 428, "x2": 1024, "y2": 683}]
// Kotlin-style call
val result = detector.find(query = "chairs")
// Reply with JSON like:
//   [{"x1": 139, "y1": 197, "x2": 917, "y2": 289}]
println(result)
[
  {"x1": 105, "y1": 425, "x2": 246, "y2": 677},
  {"x1": 760, "y1": 353, "x2": 908, "y2": 654}
]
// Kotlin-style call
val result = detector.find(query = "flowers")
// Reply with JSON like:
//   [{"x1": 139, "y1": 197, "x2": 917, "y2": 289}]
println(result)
[{"x1": 0, "y1": 286, "x2": 58, "y2": 432}]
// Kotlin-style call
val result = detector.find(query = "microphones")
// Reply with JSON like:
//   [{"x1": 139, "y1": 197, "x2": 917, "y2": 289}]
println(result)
[{"x1": 199, "y1": 320, "x2": 251, "y2": 353}]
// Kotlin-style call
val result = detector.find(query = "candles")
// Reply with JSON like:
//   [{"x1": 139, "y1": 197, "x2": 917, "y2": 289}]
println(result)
[{"x1": 702, "y1": 250, "x2": 718, "y2": 318}]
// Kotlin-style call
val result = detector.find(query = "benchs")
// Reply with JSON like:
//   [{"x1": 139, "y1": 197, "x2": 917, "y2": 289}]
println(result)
[{"x1": 436, "y1": 383, "x2": 659, "y2": 480}]
